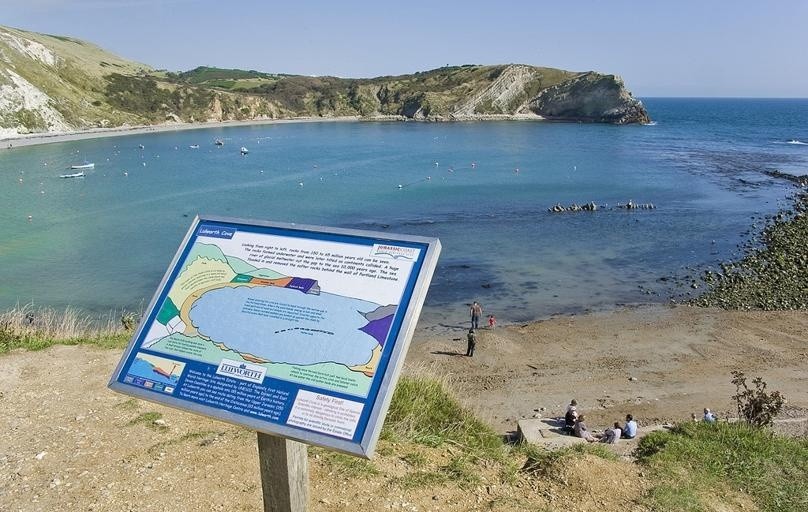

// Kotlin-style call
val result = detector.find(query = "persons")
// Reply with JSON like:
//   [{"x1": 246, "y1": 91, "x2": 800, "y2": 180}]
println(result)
[
  {"x1": 703, "y1": 408, "x2": 718, "y2": 424},
  {"x1": 565, "y1": 399, "x2": 637, "y2": 445},
  {"x1": 487, "y1": 315, "x2": 496, "y2": 330},
  {"x1": 470, "y1": 301, "x2": 482, "y2": 329},
  {"x1": 690, "y1": 412, "x2": 699, "y2": 424},
  {"x1": 464, "y1": 329, "x2": 476, "y2": 357}
]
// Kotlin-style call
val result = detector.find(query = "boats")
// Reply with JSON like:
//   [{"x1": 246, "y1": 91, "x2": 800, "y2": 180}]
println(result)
[
  {"x1": 215, "y1": 141, "x2": 223, "y2": 145},
  {"x1": 241, "y1": 146, "x2": 248, "y2": 154},
  {"x1": 189, "y1": 145, "x2": 199, "y2": 149},
  {"x1": 72, "y1": 162, "x2": 95, "y2": 169},
  {"x1": 60, "y1": 171, "x2": 84, "y2": 178}
]
